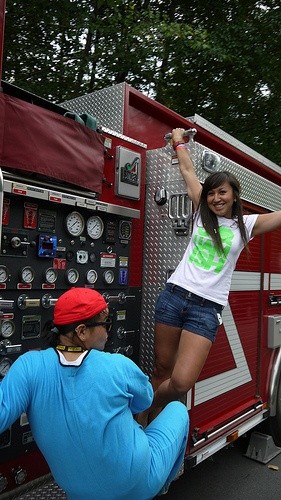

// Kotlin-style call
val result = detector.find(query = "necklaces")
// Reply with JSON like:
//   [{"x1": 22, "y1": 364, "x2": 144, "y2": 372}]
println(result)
[{"x1": 56, "y1": 344, "x2": 85, "y2": 352}]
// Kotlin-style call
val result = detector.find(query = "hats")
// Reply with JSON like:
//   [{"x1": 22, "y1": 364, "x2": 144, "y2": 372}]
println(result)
[{"x1": 52, "y1": 286, "x2": 108, "y2": 325}]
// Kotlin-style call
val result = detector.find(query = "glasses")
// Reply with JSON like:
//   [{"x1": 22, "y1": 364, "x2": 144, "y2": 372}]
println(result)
[{"x1": 86, "y1": 318, "x2": 113, "y2": 332}]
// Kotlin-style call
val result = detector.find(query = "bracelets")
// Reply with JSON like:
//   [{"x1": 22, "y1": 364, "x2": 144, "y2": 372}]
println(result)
[{"x1": 172, "y1": 140, "x2": 188, "y2": 153}]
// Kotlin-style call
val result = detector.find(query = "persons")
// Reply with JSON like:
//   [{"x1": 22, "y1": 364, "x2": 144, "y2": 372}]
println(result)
[
  {"x1": 134, "y1": 125, "x2": 281, "y2": 431},
  {"x1": 0, "y1": 286, "x2": 190, "y2": 500}
]
